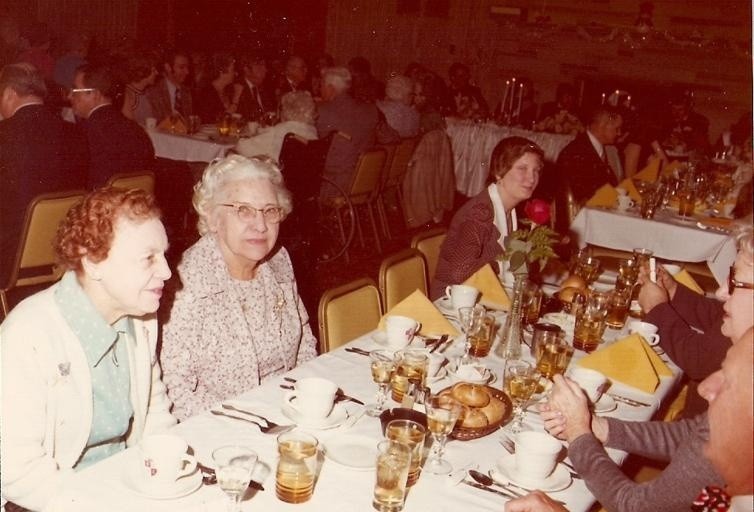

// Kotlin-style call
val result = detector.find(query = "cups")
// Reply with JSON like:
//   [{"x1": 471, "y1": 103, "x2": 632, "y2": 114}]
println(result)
[
  {"x1": 145, "y1": 118, "x2": 156, "y2": 128},
  {"x1": 270, "y1": 245, "x2": 661, "y2": 512},
  {"x1": 617, "y1": 196, "x2": 634, "y2": 208},
  {"x1": 637, "y1": 163, "x2": 733, "y2": 220},
  {"x1": 134, "y1": 434, "x2": 196, "y2": 487},
  {"x1": 188, "y1": 114, "x2": 200, "y2": 133},
  {"x1": 218, "y1": 114, "x2": 259, "y2": 138}
]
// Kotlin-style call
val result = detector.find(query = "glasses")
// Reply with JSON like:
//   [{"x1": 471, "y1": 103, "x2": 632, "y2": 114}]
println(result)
[
  {"x1": 726, "y1": 260, "x2": 754, "y2": 295},
  {"x1": 216, "y1": 203, "x2": 283, "y2": 223}
]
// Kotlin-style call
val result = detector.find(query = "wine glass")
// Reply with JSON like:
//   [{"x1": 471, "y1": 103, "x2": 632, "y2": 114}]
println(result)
[{"x1": 211, "y1": 445, "x2": 258, "y2": 511}]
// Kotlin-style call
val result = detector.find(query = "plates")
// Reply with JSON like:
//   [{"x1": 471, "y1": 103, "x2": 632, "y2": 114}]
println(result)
[{"x1": 121, "y1": 458, "x2": 204, "y2": 499}]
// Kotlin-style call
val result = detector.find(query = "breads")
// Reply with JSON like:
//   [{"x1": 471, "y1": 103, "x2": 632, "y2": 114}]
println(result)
[
  {"x1": 437, "y1": 382, "x2": 507, "y2": 428},
  {"x1": 558, "y1": 275, "x2": 593, "y2": 304}
]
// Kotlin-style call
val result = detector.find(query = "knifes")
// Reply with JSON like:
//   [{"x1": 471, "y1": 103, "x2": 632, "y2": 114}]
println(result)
[{"x1": 197, "y1": 462, "x2": 263, "y2": 491}]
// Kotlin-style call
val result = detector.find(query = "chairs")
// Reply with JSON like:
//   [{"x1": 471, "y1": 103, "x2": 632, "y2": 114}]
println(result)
[{"x1": 0, "y1": 115, "x2": 753, "y2": 512}]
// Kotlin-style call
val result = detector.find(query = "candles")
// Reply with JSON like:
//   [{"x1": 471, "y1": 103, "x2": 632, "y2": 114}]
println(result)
[{"x1": 500, "y1": 75, "x2": 633, "y2": 115}]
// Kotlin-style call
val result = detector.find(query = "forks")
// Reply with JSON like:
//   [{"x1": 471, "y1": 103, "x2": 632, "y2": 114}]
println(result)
[
  {"x1": 696, "y1": 222, "x2": 730, "y2": 233},
  {"x1": 210, "y1": 403, "x2": 294, "y2": 434}
]
